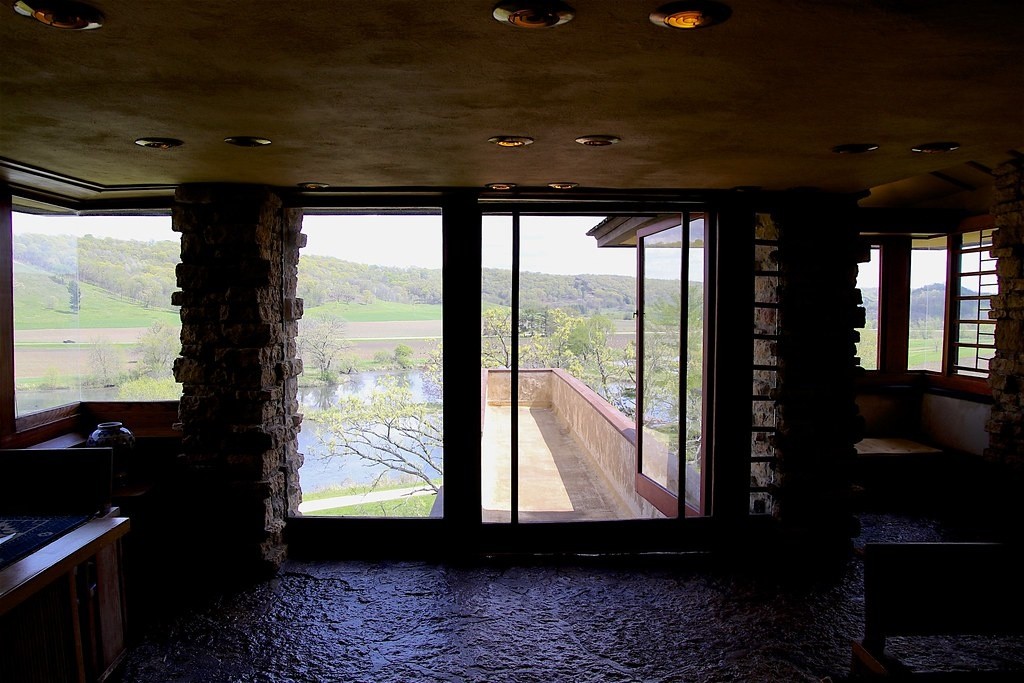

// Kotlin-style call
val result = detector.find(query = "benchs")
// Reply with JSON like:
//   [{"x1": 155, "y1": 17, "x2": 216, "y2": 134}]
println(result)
[
  {"x1": 18, "y1": 397, "x2": 182, "y2": 448},
  {"x1": 851, "y1": 540, "x2": 1023, "y2": 683}
]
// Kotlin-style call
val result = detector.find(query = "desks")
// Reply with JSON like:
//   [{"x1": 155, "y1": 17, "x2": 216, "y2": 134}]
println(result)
[{"x1": 0, "y1": 516, "x2": 131, "y2": 683}]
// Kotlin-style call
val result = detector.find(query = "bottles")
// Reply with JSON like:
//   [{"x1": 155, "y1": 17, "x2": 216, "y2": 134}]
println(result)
[
  {"x1": 82, "y1": 560, "x2": 98, "y2": 598},
  {"x1": 87, "y1": 422, "x2": 137, "y2": 484}
]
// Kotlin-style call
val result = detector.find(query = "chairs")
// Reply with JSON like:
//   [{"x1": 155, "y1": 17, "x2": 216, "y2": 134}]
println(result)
[{"x1": 0, "y1": 446, "x2": 120, "y2": 683}]
